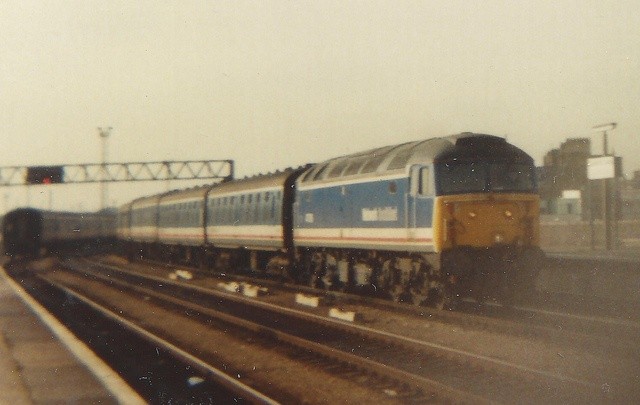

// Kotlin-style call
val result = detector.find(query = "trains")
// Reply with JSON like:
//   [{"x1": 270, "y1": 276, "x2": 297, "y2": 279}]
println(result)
[
  {"x1": 117, "y1": 131, "x2": 541, "y2": 311},
  {"x1": 2, "y1": 207, "x2": 117, "y2": 255}
]
